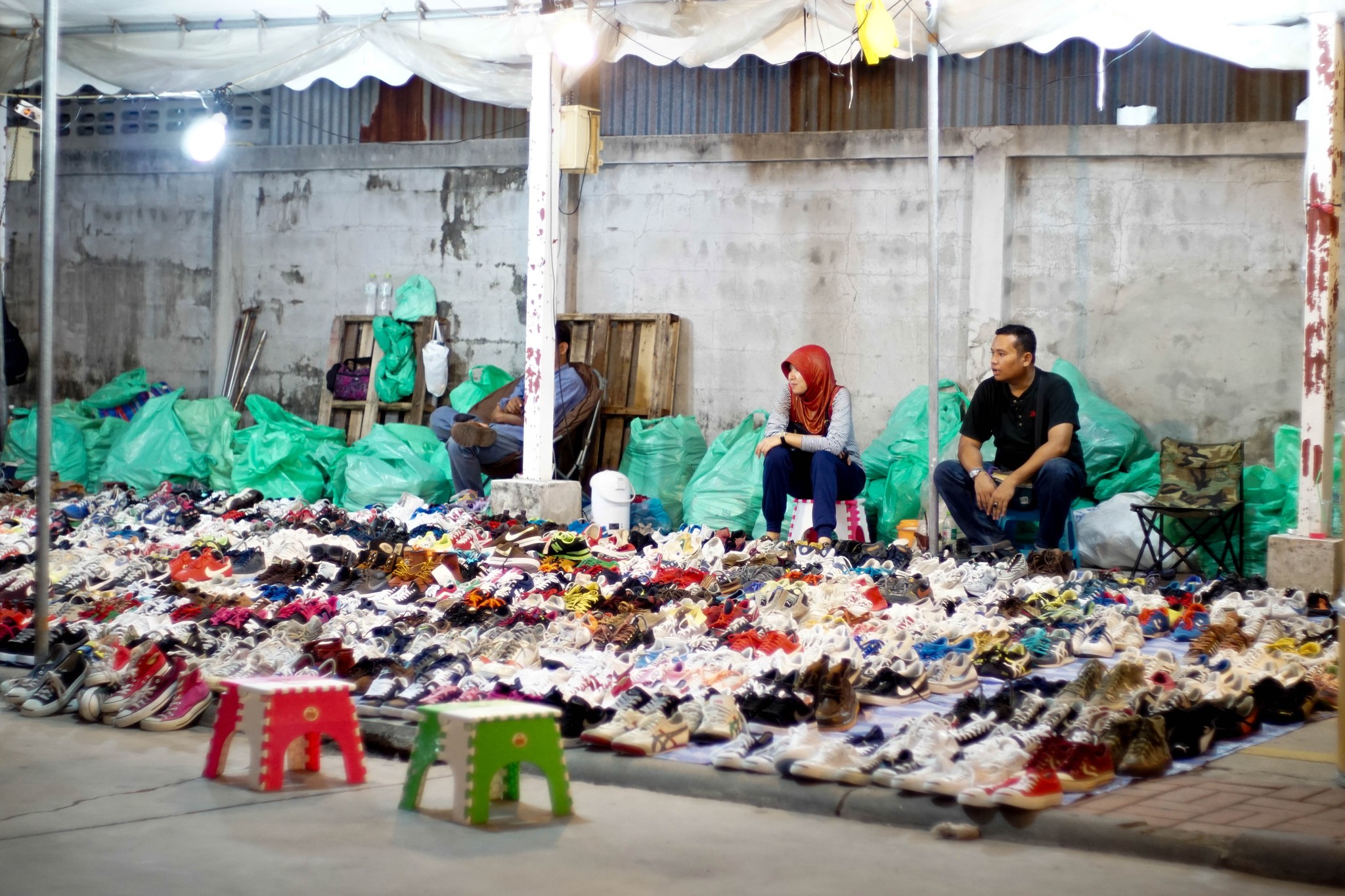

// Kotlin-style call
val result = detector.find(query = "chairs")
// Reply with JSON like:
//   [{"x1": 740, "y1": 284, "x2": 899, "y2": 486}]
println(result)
[
  {"x1": 1121, "y1": 437, "x2": 1249, "y2": 587},
  {"x1": 469, "y1": 362, "x2": 605, "y2": 512}
]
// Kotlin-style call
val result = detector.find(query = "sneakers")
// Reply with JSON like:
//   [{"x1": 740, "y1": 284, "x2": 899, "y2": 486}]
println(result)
[{"x1": 0, "y1": 460, "x2": 1340, "y2": 812}]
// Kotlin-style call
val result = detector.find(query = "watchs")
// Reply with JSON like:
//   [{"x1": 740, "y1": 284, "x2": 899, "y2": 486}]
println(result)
[
  {"x1": 969, "y1": 468, "x2": 985, "y2": 481},
  {"x1": 779, "y1": 431, "x2": 787, "y2": 444}
]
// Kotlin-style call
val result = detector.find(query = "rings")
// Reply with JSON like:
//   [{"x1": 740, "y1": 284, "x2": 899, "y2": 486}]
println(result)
[{"x1": 993, "y1": 503, "x2": 998, "y2": 508}]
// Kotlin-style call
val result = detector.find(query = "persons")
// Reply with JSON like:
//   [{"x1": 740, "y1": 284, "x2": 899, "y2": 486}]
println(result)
[
  {"x1": 754, "y1": 345, "x2": 866, "y2": 543},
  {"x1": 934, "y1": 324, "x2": 1088, "y2": 559},
  {"x1": 428, "y1": 319, "x2": 592, "y2": 497}
]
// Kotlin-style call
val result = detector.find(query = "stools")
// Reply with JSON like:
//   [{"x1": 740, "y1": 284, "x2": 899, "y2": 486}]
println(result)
[
  {"x1": 398, "y1": 698, "x2": 577, "y2": 825},
  {"x1": 786, "y1": 496, "x2": 872, "y2": 549},
  {"x1": 992, "y1": 508, "x2": 1081, "y2": 572},
  {"x1": 197, "y1": 678, "x2": 368, "y2": 793}
]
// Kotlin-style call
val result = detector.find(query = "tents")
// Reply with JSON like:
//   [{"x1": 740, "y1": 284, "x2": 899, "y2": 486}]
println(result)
[{"x1": 3, "y1": 0, "x2": 1345, "y2": 535}]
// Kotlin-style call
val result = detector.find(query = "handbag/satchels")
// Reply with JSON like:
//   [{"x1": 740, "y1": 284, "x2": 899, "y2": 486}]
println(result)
[
  {"x1": 332, "y1": 357, "x2": 372, "y2": 399},
  {"x1": 422, "y1": 320, "x2": 449, "y2": 398}
]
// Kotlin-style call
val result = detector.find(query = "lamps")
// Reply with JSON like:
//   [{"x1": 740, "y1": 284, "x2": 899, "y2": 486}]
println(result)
[{"x1": 179, "y1": 82, "x2": 237, "y2": 162}]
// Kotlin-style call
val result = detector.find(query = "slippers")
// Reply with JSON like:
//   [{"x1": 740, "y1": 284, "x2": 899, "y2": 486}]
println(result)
[{"x1": 449, "y1": 421, "x2": 496, "y2": 448}]
